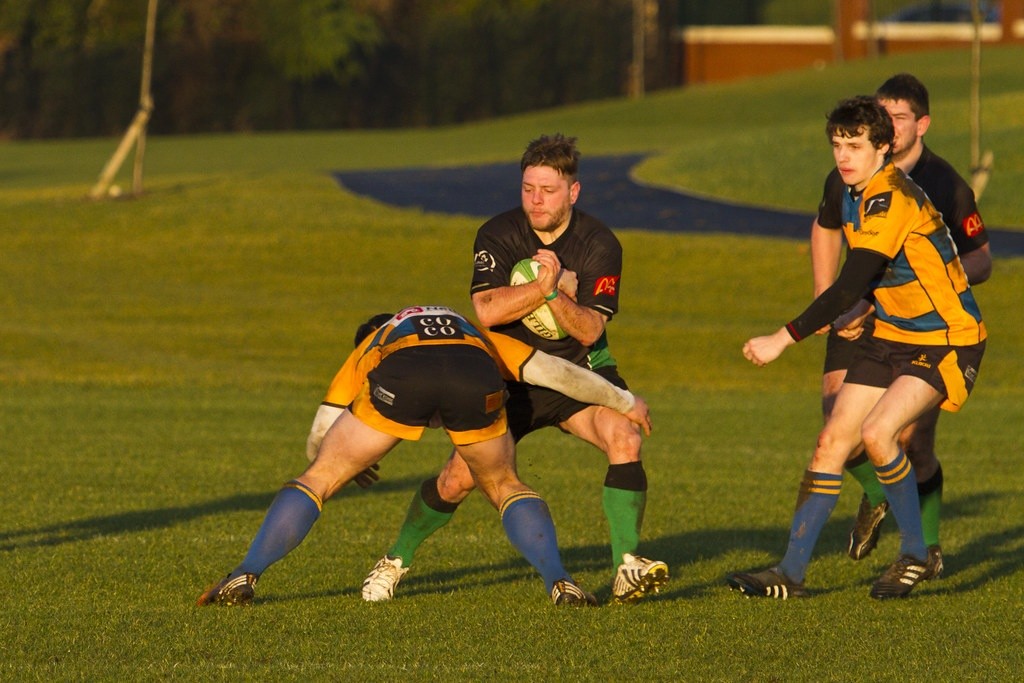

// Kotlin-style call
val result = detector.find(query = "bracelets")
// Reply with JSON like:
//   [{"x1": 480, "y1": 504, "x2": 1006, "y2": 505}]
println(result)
[{"x1": 545, "y1": 289, "x2": 557, "y2": 301}]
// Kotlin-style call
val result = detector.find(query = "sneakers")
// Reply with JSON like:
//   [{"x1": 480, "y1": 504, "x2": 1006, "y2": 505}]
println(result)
[
  {"x1": 870, "y1": 549, "x2": 935, "y2": 600},
  {"x1": 198, "y1": 572, "x2": 257, "y2": 606},
  {"x1": 848, "y1": 492, "x2": 890, "y2": 560},
  {"x1": 613, "y1": 553, "x2": 670, "y2": 605},
  {"x1": 551, "y1": 579, "x2": 597, "y2": 608},
  {"x1": 361, "y1": 555, "x2": 409, "y2": 601},
  {"x1": 924, "y1": 544, "x2": 944, "y2": 579},
  {"x1": 727, "y1": 566, "x2": 810, "y2": 598}
]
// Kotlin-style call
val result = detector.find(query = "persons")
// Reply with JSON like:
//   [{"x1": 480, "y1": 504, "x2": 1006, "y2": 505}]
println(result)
[
  {"x1": 362, "y1": 132, "x2": 670, "y2": 607},
  {"x1": 195, "y1": 303, "x2": 652, "y2": 608},
  {"x1": 724, "y1": 95, "x2": 988, "y2": 602},
  {"x1": 808, "y1": 74, "x2": 993, "y2": 582}
]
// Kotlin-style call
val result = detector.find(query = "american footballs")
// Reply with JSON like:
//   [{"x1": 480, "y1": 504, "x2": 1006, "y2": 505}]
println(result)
[{"x1": 508, "y1": 257, "x2": 569, "y2": 341}]
